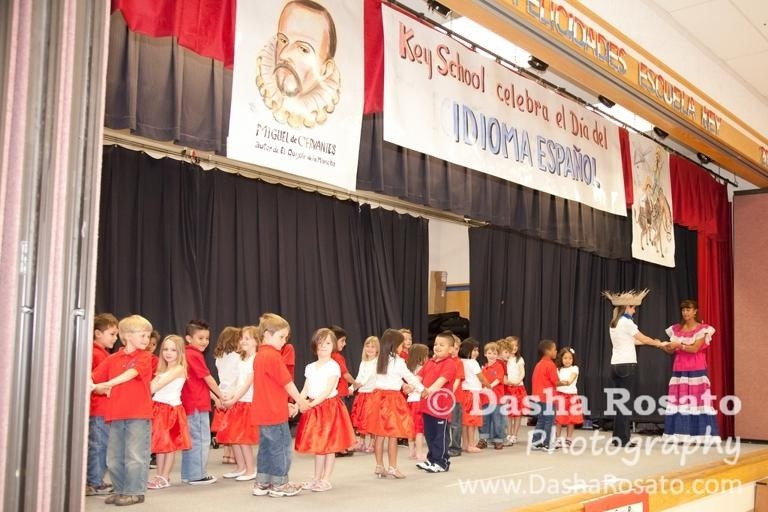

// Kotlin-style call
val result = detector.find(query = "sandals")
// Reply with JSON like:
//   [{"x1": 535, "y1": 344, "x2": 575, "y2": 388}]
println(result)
[
  {"x1": 555, "y1": 440, "x2": 562, "y2": 449},
  {"x1": 222, "y1": 454, "x2": 237, "y2": 464},
  {"x1": 313, "y1": 481, "x2": 333, "y2": 492},
  {"x1": 304, "y1": 478, "x2": 319, "y2": 488},
  {"x1": 150, "y1": 475, "x2": 172, "y2": 490},
  {"x1": 565, "y1": 439, "x2": 572, "y2": 448}
]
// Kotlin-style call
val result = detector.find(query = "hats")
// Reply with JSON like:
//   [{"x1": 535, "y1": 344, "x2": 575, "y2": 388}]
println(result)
[{"x1": 603, "y1": 287, "x2": 651, "y2": 306}]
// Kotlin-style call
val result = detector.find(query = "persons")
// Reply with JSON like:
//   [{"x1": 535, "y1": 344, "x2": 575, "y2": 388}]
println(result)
[
  {"x1": 475, "y1": 343, "x2": 505, "y2": 449},
  {"x1": 661, "y1": 300, "x2": 721, "y2": 446},
  {"x1": 349, "y1": 336, "x2": 380, "y2": 452},
  {"x1": 555, "y1": 346, "x2": 579, "y2": 449},
  {"x1": 181, "y1": 320, "x2": 228, "y2": 486},
  {"x1": 222, "y1": 327, "x2": 258, "y2": 481},
  {"x1": 371, "y1": 328, "x2": 429, "y2": 479},
  {"x1": 407, "y1": 343, "x2": 430, "y2": 461},
  {"x1": 507, "y1": 336, "x2": 531, "y2": 445},
  {"x1": 148, "y1": 331, "x2": 160, "y2": 378},
  {"x1": 403, "y1": 333, "x2": 457, "y2": 473},
  {"x1": 399, "y1": 328, "x2": 412, "y2": 364},
  {"x1": 448, "y1": 333, "x2": 466, "y2": 460},
  {"x1": 331, "y1": 325, "x2": 359, "y2": 457},
  {"x1": 251, "y1": 313, "x2": 303, "y2": 497},
  {"x1": 459, "y1": 337, "x2": 491, "y2": 454},
  {"x1": 210, "y1": 326, "x2": 241, "y2": 465},
  {"x1": 85, "y1": 313, "x2": 114, "y2": 496},
  {"x1": 88, "y1": 382, "x2": 109, "y2": 396},
  {"x1": 257, "y1": 0, "x2": 341, "y2": 126},
  {"x1": 532, "y1": 339, "x2": 563, "y2": 450},
  {"x1": 603, "y1": 289, "x2": 670, "y2": 448},
  {"x1": 295, "y1": 327, "x2": 358, "y2": 492},
  {"x1": 93, "y1": 315, "x2": 154, "y2": 506},
  {"x1": 280, "y1": 329, "x2": 296, "y2": 430},
  {"x1": 497, "y1": 339, "x2": 512, "y2": 445},
  {"x1": 146, "y1": 335, "x2": 193, "y2": 490}
]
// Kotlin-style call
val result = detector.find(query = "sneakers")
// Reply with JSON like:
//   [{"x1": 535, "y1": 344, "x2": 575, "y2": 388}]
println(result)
[
  {"x1": 417, "y1": 460, "x2": 432, "y2": 470},
  {"x1": 477, "y1": 440, "x2": 488, "y2": 448},
  {"x1": 427, "y1": 463, "x2": 448, "y2": 472},
  {"x1": 467, "y1": 446, "x2": 481, "y2": 453},
  {"x1": 87, "y1": 484, "x2": 114, "y2": 495},
  {"x1": 189, "y1": 475, "x2": 218, "y2": 485},
  {"x1": 252, "y1": 483, "x2": 272, "y2": 495},
  {"x1": 269, "y1": 483, "x2": 304, "y2": 497},
  {"x1": 495, "y1": 443, "x2": 504, "y2": 450},
  {"x1": 510, "y1": 436, "x2": 517, "y2": 444}
]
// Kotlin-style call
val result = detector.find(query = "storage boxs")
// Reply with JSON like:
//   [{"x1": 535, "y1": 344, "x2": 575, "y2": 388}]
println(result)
[{"x1": 428, "y1": 271, "x2": 449, "y2": 315}]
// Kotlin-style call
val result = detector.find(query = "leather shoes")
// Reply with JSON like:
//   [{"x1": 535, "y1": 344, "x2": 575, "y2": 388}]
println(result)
[
  {"x1": 105, "y1": 494, "x2": 119, "y2": 502},
  {"x1": 388, "y1": 468, "x2": 405, "y2": 480},
  {"x1": 222, "y1": 471, "x2": 245, "y2": 479},
  {"x1": 235, "y1": 473, "x2": 257, "y2": 483},
  {"x1": 335, "y1": 449, "x2": 352, "y2": 457},
  {"x1": 615, "y1": 442, "x2": 635, "y2": 447},
  {"x1": 114, "y1": 494, "x2": 146, "y2": 505},
  {"x1": 375, "y1": 466, "x2": 388, "y2": 475}
]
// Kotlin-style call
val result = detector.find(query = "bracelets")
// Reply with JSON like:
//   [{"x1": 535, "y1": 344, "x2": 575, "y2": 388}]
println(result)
[{"x1": 682, "y1": 345, "x2": 687, "y2": 353}]
